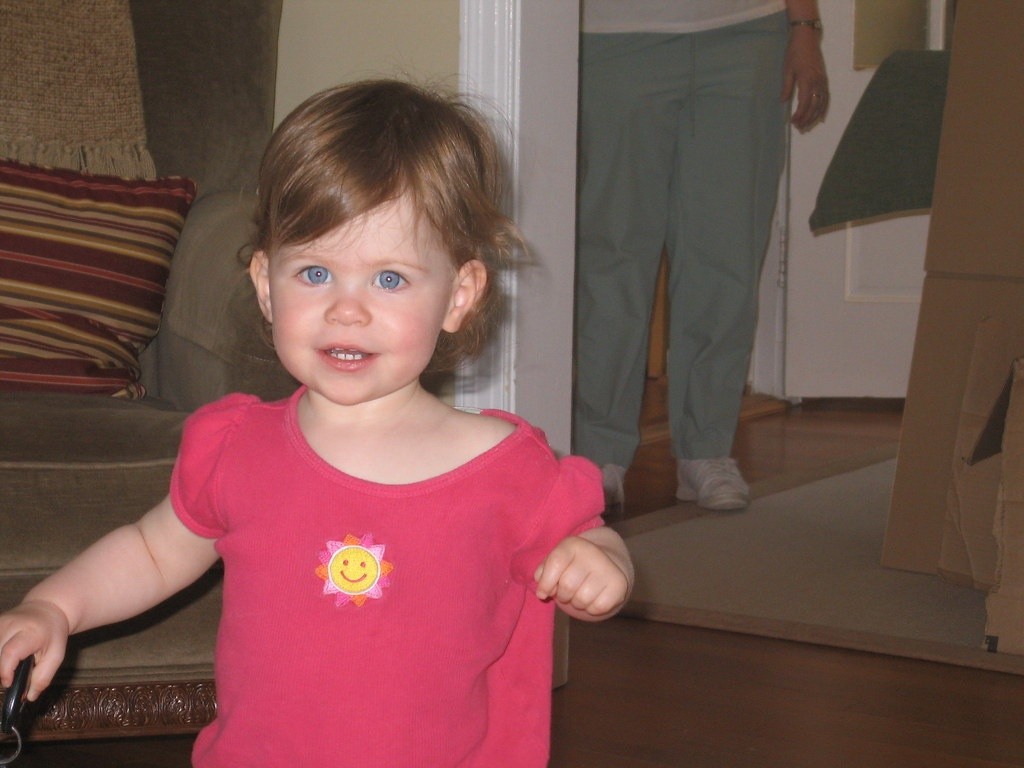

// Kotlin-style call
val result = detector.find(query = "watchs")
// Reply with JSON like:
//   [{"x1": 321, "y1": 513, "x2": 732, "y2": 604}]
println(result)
[{"x1": 791, "y1": 19, "x2": 822, "y2": 30}]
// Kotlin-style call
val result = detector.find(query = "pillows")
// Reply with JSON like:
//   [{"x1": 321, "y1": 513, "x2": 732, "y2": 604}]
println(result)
[{"x1": 0, "y1": 159, "x2": 198, "y2": 400}]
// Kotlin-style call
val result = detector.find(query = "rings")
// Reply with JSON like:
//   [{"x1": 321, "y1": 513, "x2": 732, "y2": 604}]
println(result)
[{"x1": 812, "y1": 94, "x2": 821, "y2": 98}]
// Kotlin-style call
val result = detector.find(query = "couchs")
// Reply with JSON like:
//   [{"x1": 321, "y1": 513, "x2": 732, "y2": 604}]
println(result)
[{"x1": 0, "y1": 0, "x2": 285, "y2": 562}]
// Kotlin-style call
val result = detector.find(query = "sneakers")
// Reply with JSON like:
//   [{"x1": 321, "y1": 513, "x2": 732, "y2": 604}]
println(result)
[
  {"x1": 601, "y1": 464, "x2": 628, "y2": 514},
  {"x1": 675, "y1": 456, "x2": 749, "y2": 510}
]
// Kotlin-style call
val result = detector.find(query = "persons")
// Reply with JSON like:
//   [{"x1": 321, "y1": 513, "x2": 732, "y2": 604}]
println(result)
[
  {"x1": 1, "y1": 79, "x2": 635, "y2": 765},
  {"x1": 574, "y1": 0, "x2": 830, "y2": 511}
]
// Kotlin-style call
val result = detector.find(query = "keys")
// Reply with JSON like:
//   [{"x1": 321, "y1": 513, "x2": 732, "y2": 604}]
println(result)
[{"x1": 0, "y1": 654, "x2": 35, "y2": 764}]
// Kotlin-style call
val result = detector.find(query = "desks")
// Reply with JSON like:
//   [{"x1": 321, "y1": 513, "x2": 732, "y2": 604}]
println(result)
[{"x1": 0, "y1": 565, "x2": 226, "y2": 744}]
